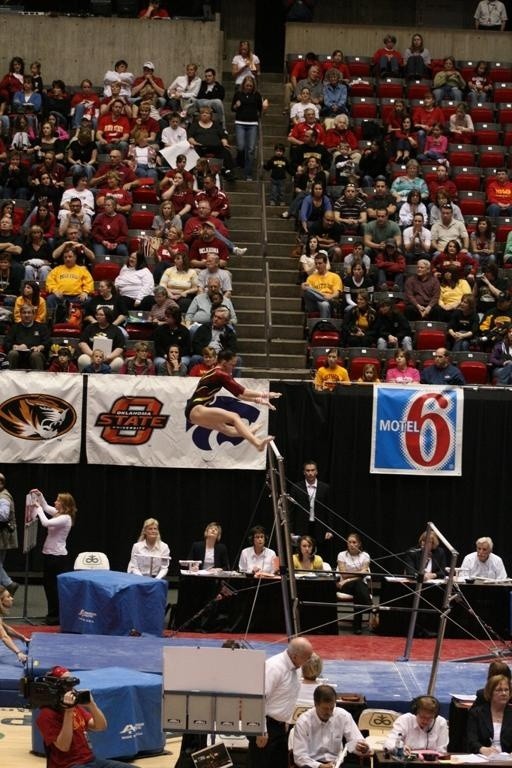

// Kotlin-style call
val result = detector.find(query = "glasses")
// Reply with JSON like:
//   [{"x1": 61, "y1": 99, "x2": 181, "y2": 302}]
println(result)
[
  {"x1": 197, "y1": 206, "x2": 209, "y2": 210},
  {"x1": 346, "y1": 184, "x2": 356, "y2": 188},
  {"x1": 442, "y1": 203, "x2": 451, "y2": 209}
]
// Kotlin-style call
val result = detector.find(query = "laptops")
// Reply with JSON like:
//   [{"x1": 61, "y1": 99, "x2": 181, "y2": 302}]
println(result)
[{"x1": 191, "y1": 742, "x2": 234, "y2": 768}]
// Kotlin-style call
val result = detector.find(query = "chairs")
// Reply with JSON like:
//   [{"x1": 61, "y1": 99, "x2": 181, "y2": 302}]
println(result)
[
  {"x1": 336, "y1": 565, "x2": 375, "y2": 633},
  {"x1": 286, "y1": 721, "x2": 348, "y2": 767},
  {"x1": 2, "y1": 72, "x2": 240, "y2": 381},
  {"x1": 288, "y1": 49, "x2": 510, "y2": 384}
]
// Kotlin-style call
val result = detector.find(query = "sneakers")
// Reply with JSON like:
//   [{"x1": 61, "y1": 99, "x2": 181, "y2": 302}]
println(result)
[{"x1": 233, "y1": 247, "x2": 249, "y2": 258}]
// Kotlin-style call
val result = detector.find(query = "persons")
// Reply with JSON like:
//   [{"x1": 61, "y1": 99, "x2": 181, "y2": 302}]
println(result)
[
  {"x1": 288, "y1": 684, "x2": 371, "y2": 768},
  {"x1": 0, "y1": 54, "x2": 233, "y2": 192},
  {"x1": 282, "y1": 34, "x2": 512, "y2": 235},
  {"x1": 386, "y1": 348, "x2": 419, "y2": 383},
  {"x1": 504, "y1": 231, "x2": 512, "y2": 266},
  {"x1": 404, "y1": 258, "x2": 439, "y2": 323},
  {"x1": 291, "y1": 534, "x2": 323, "y2": 574},
  {"x1": 38, "y1": 667, "x2": 138, "y2": 768},
  {"x1": 346, "y1": 257, "x2": 372, "y2": 336},
  {"x1": 178, "y1": 520, "x2": 230, "y2": 572},
  {"x1": 248, "y1": 637, "x2": 313, "y2": 768},
  {"x1": 0, "y1": 472, "x2": 18, "y2": 596},
  {"x1": 473, "y1": 0, "x2": 506, "y2": 31},
  {"x1": 373, "y1": 298, "x2": 410, "y2": 357},
  {"x1": 341, "y1": 290, "x2": 377, "y2": 358},
  {"x1": 285, "y1": 653, "x2": 337, "y2": 739},
  {"x1": 466, "y1": 675, "x2": 512, "y2": 755},
  {"x1": 314, "y1": 350, "x2": 351, "y2": 388},
  {"x1": 238, "y1": 526, "x2": 279, "y2": 577},
  {"x1": 30, "y1": 489, "x2": 76, "y2": 626},
  {"x1": 289, "y1": 460, "x2": 332, "y2": 544},
  {"x1": 335, "y1": 534, "x2": 380, "y2": 633},
  {"x1": 1, "y1": 587, "x2": 33, "y2": 663},
  {"x1": 462, "y1": 535, "x2": 507, "y2": 577},
  {"x1": 297, "y1": 252, "x2": 341, "y2": 322},
  {"x1": 261, "y1": 141, "x2": 290, "y2": 206},
  {"x1": 443, "y1": 265, "x2": 469, "y2": 317},
  {"x1": 232, "y1": 38, "x2": 262, "y2": 98},
  {"x1": 470, "y1": 218, "x2": 500, "y2": 282},
  {"x1": 357, "y1": 364, "x2": 381, "y2": 382},
  {"x1": 431, "y1": 240, "x2": 479, "y2": 281},
  {"x1": 471, "y1": 264, "x2": 508, "y2": 314},
  {"x1": 128, "y1": 517, "x2": 170, "y2": 582},
  {"x1": 479, "y1": 292, "x2": 512, "y2": 348},
  {"x1": 388, "y1": 696, "x2": 451, "y2": 756},
  {"x1": 404, "y1": 531, "x2": 450, "y2": 578},
  {"x1": 231, "y1": 76, "x2": 262, "y2": 181},
  {"x1": 184, "y1": 348, "x2": 283, "y2": 453},
  {"x1": 374, "y1": 238, "x2": 407, "y2": 292},
  {"x1": 422, "y1": 348, "x2": 467, "y2": 385},
  {"x1": 140, "y1": 0, "x2": 173, "y2": 20},
  {"x1": 0, "y1": 174, "x2": 247, "y2": 374},
  {"x1": 299, "y1": 236, "x2": 330, "y2": 282},
  {"x1": 464, "y1": 660, "x2": 512, "y2": 711},
  {"x1": 450, "y1": 294, "x2": 482, "y2": 360},
  {"x1": 489, "y1": 325, "x2": 512, "y2": 388},
  {"x1": 344, "y1": 243, "x2": 370, "y2": 275}
]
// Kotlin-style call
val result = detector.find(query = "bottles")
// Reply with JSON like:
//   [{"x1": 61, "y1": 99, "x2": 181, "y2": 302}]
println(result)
[{"x1": 393, "y1": 732, "x2": 406, "y2": 763}]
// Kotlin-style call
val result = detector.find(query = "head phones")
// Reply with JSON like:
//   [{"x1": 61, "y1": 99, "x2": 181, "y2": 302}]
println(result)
[{"x1": 410, "y1": 695, "x2": 442, "y2": 717}]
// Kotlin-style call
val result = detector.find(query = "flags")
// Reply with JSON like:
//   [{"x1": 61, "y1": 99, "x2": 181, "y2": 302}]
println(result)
[
  {"x1": 87, "y1": 374, "x2": 267, "y2": 470},
  {"x1": 1, "y1": 371, "x2": 83, "y2": 464},
  {"x1": 369, "y1": 383, "x2": 462, "y2": 476}
]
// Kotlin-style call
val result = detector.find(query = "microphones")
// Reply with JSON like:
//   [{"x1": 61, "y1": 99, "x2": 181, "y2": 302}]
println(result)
[{"x1": 426, "y1": 717, "x2": 438, "y2": 733}]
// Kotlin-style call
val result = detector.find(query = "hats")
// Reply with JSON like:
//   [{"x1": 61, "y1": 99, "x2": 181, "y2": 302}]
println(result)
[
  {"x1": 203, "y1": 221, "x2": 214, "y2": 229},
  {"x1": 143, "y1": 61, "x2": 155, "y2": 70},
  {"x1": 384, "y1": 238, "x2": 397, "y2": 249}
]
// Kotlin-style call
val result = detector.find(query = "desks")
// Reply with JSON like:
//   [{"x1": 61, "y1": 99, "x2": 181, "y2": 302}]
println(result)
[
  {"x1": 379, "y1": 575, "x2": 512, "y2": 641},
  {"x1": 336, "y1": 692, "x2": 367, "y2": 739},
  {"x1": 450, "y1": 694, "x2": 480, "y2": 753},
  {"x1": 176, "y1": 568, "x2": 339, "y2": 637}
]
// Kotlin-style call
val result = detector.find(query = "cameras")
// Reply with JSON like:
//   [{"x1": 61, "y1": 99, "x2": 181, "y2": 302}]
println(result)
[{"x1": 33, "y1": 674, "x2": 91, "y2": 709}]
